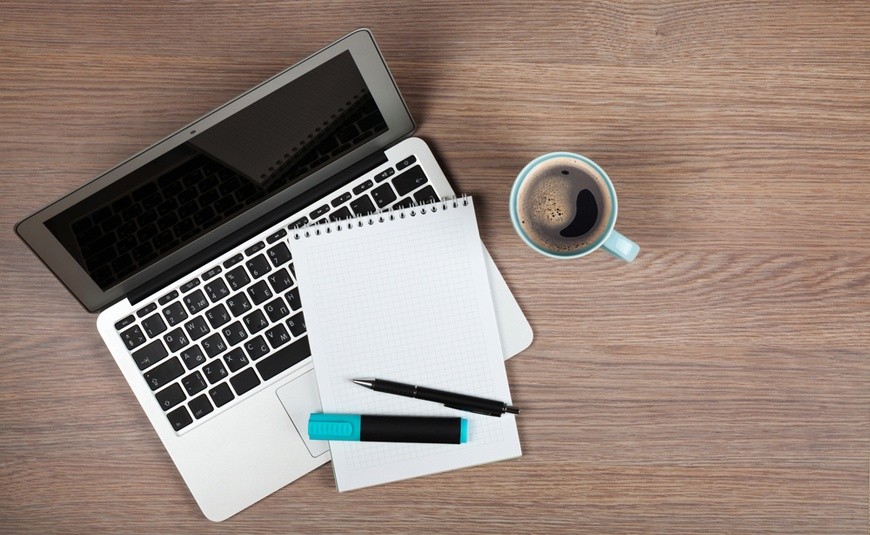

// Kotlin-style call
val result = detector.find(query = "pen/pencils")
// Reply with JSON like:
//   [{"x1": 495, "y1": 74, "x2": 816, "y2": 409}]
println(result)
[{"x1": 353, "y1": 378, "x2": 521, "y2": 417}]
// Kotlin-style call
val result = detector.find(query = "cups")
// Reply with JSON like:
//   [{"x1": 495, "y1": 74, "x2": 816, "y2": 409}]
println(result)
[{"x1": 508, "y1": 151, "x2": 640, "y2": 263}]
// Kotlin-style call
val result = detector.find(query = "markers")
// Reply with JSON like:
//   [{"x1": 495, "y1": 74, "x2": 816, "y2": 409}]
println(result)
[{"x1": 308, "y1": 413, "x2": 468, "y2": 445}]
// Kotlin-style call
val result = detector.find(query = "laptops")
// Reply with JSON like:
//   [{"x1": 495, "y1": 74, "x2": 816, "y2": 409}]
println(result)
[
  {"x1": 13, "y1": 28, "x2": 533, "y2": 523},
  {"x1": 72, "y1": 91, "x2": 388, "y2": 293}
]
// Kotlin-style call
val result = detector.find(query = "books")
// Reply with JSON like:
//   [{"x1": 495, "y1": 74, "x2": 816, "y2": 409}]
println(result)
[{"x1": 288, "y1": 192, "x2": 523, "y2": 494}]
